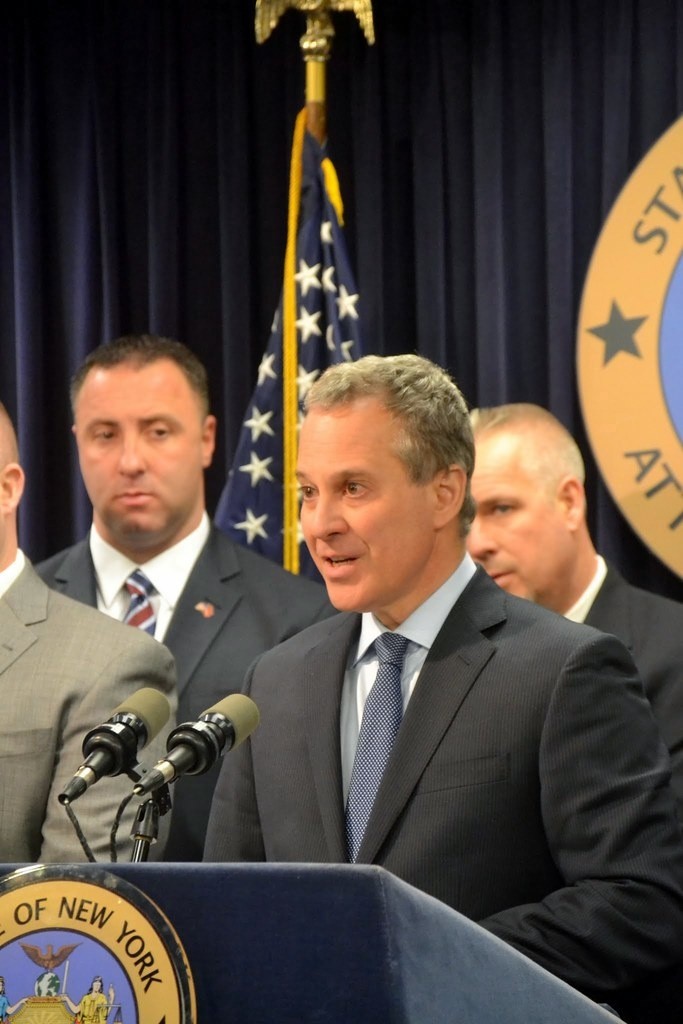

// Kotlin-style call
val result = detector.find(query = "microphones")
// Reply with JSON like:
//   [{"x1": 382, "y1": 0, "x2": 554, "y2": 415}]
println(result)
[
  {"x1": 133, "y1": 693, "x2": 260, "y2": 796},
  {"x1": 57, "y1": 687, "x2": 170, "y2": 806}
]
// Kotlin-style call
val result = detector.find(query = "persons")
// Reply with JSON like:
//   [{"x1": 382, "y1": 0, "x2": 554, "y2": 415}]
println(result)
[
  {"x1": 197, "y1": 350, "x2": 683, "y2": 1024},
  {"x1": 28, "y1": 339, "x2": 337, "y2": 866},
  {"x1": 0, "y1": 400, "x2": 182, "y2": 865},
  {"x1": 467, "y1": 399, "x2": 683, "y2": 806}
]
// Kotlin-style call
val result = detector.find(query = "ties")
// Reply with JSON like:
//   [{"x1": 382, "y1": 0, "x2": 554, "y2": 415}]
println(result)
[
  {"x1": 122, "y1": 569, "x2": 157, "y2": 636},
  {"x1": 344, "y1": 631, "x2": 409, "y2": 863}
]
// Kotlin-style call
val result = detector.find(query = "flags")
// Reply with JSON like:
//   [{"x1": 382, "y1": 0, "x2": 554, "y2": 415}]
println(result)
[{"x1": 208, "y1": 101, "x2": 361, "y2": 588}]
